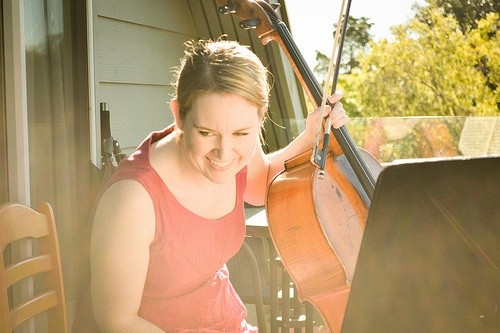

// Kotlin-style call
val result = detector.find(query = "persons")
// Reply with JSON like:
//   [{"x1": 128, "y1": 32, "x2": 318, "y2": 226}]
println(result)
[{"x1": 71, "y1": 35, "x2": 349, "y2": 333}]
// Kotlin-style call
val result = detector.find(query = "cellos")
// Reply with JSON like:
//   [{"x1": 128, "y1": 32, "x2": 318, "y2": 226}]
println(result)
[{"x1": 219, "y1": 0, "x2": 385, "y2": 332}]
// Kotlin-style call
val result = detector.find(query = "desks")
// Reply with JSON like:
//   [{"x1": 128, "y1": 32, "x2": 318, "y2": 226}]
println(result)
[{"x1": 240, "y1": 207, "x2": 314, "y2": 332}]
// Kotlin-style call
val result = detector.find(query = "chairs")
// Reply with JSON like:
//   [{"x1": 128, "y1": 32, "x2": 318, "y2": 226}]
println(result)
[{"x1": 0, "y1": 202, "x2": 69, "y2": 333}]
